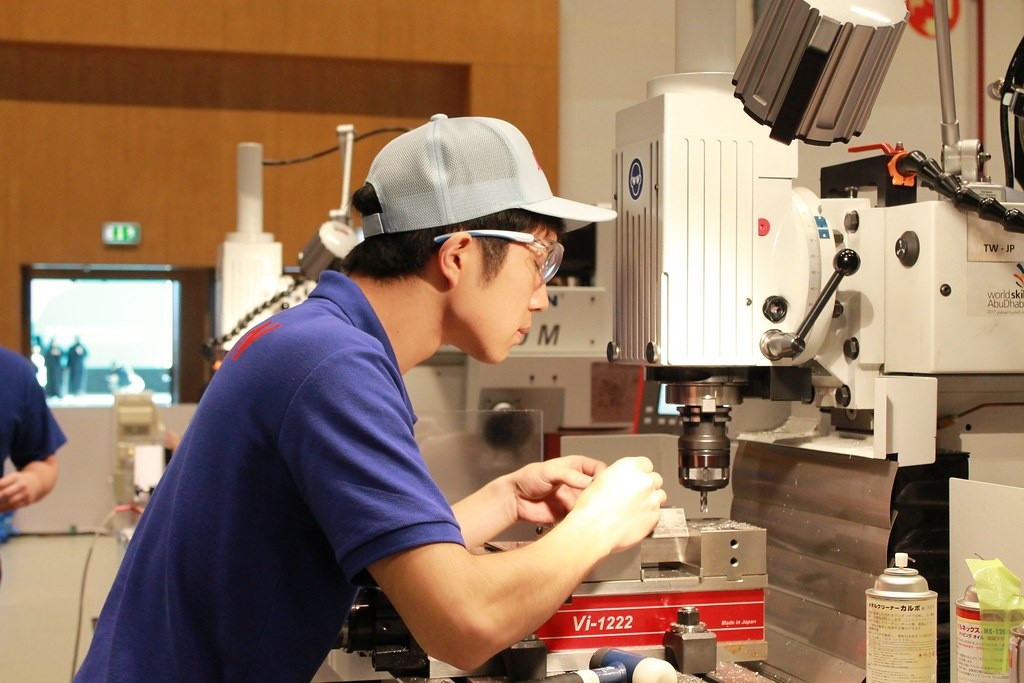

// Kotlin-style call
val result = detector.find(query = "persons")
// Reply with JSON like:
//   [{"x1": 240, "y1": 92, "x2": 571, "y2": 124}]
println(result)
[
  {"x1": 75, "y1": 114, "x2": 667, "y2": 683},
  {"x1": 0, "y1": 347, "x2": 67, "y2": 549},
  {"x1": 30, "y1": 334, "x2": 89, "y2": 399}
]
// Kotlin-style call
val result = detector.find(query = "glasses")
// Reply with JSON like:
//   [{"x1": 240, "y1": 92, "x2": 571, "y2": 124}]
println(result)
[{"x1": 434, "y1": 229, "x2": 565, "y2": 284}]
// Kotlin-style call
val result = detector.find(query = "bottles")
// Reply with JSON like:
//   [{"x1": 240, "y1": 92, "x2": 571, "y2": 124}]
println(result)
[
  {"x1": 864, "y1": 553, "x2": 938, "y2": 683},
  {"x1": 1008, "y1": 621, "x2": 1024, "y2": 683},
  {"x1": 955, "y1": 565, "x2": 1024, "y2": 682}
]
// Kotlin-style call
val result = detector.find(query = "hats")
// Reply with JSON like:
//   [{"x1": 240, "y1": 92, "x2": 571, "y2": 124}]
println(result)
[{"x1": 361, "y1": 113, "x2": 618, "y2": 238}]
